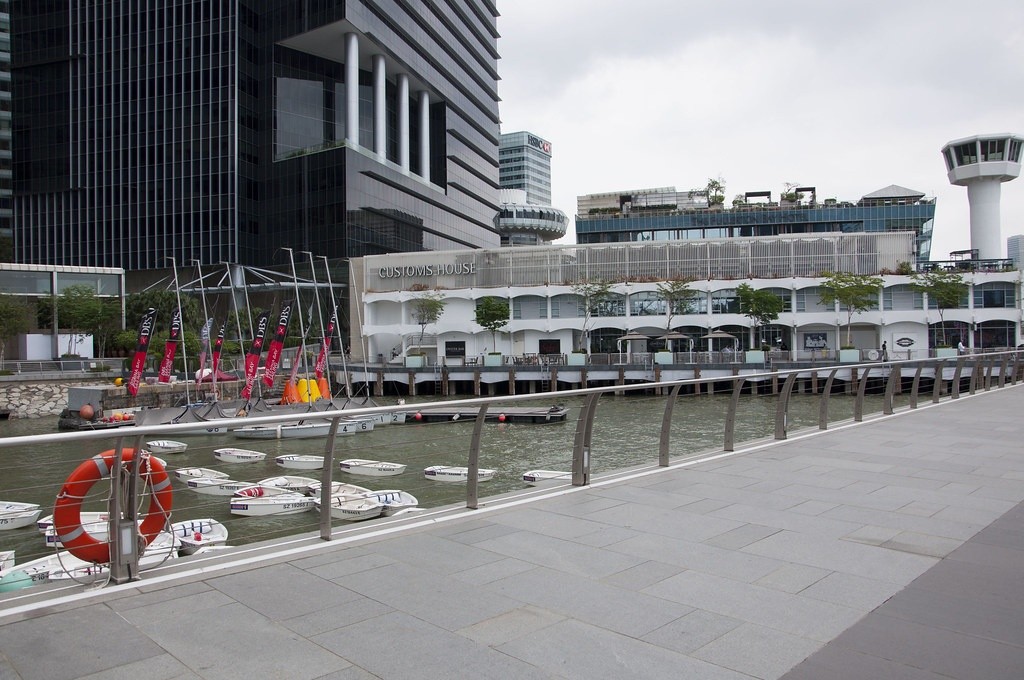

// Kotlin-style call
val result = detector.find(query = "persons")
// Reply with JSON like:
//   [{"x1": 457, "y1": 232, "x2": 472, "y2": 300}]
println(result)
[
  {"x1": 958, "y1": 340, "x2": 964, "y2": 349},
  {"x1": 391, "y1": 348, "x2": 398, "y2": 359},
  {"x1": 882, "y1": 341, "x2": 886, "y2": 355}
]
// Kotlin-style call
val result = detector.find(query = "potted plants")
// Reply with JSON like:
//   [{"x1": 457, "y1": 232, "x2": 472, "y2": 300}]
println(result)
[
  {"x1": 906, "y1": 200, "x2": 913, "y2": 206},
  {"x1": 824, "y1": 196, "x2": 837, "y2": 203},
  {"x1": 919, "y1": 199, "x2": 927, "y2": 204},
  {"x1": 780, "y1": 181, "x2": 799, "y2": 207},
  {"x1": 762, "y1": 202, "x2": 778, "y2": 207},
  {"x1": 588, "y1": 208, "x2": 599, "y2": 215},
  {"x1": 797, "y1": 193, "x2": 803, "y2": 207},
  {"x1": 609, "y1": 207, "x2": 621, "y2": 215},
  {"x1": 877, "y1": 201, "x2": 883, "y2": 206},
  {"x1": 732, "y1": 195, "x2": 744, "y2": 208},
  {"x1": 708, "y1": 178, "x2": 726, "y2": 209},
  {"x1": 600, "y1": 207, "x2": 609, "y2": 214}
]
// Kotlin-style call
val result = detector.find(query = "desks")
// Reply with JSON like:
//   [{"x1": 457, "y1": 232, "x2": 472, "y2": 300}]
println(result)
[{"x1": 547, "y1": 354, "x2": 561, "y2": 365}]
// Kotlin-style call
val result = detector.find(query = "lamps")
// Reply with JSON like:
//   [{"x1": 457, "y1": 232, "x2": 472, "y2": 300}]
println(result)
[
  {"x1": 973, "y1": 321, "x2": 977, "y2": 331},
  {"x1": 794, "y1": 325, "x2": 796, "y2": 334},
  {"x1": 585, "y1": 328, "x2": 589, "y2": 338}
]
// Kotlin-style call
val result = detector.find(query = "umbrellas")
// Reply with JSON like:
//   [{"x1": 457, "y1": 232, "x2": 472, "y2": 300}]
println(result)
[
  {"x1": 656, "y1": 331, "x2": 690, "y2": 352},
  {"x1": 617, "y1": 331, "x2": 652, "y2": 353},
  {"x1": 700, "y1": 330, "x2": 736, "y2": 351}
]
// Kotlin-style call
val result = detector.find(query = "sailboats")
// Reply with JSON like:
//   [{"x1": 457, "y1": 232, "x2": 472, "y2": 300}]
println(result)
[{"x1": 133, "y1": 247, "x2": 407, "y2": 439}]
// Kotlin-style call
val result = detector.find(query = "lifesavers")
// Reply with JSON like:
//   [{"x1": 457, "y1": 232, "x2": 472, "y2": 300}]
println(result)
[{"x1": 54, "y1": 449, "x2": 172, "y2": 563}]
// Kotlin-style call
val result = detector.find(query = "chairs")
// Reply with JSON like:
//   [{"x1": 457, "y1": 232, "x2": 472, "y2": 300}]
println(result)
[
  {"x1": 539, "y1": 355, "x2": 548, "y2": 364},
  {"x1": 558, "y1": 353, "x2": 565, "y2": 365},
  {"x1": 512, "y1": 357, "x2": 522, "y2": 365},
  {"x1": 506, "y1": 357, "x2": 510, "y2": 363},
  {"x1": 470, "y1": 357, "x2": 478, "y2": 365}
]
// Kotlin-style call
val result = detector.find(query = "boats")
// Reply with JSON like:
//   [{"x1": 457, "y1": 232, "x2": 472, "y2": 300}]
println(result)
[
  {"x1": 424, "y1": 464, "x2": 497, "y2": 483},
  {"x1": 146, "y1": 438, "x2": 188, "y2": 454},
  {"x1": 214, "y1": 446, "x2": 268, "y2": 464},
  {"x1": 0, "y1": 499, "x2": 184, "y2": 593},
  {"x1": 340, "y1": 457, "x2": 406, "y2": 477},
  {"x1": 229, "y1": 475, "x2": 419, "y2": 522},
  {"x1": 275, "y1": 452, "x2": 336, "y2": 470},
  {"x1": 174, "y1": 466, "x2": 231, "y2": 485},
  {"x1": 186, "y1": 476, "x2": 254, "y2": 495},
  {"x1": 169, "y1": 517, "x2": 229, "y2": 555},
  {"x1": 524, "y1": 469, "x2": 574, "y2": 486},
  {"x1": 152, "y1": 455, "x2": 168, "y2": 472}
]
